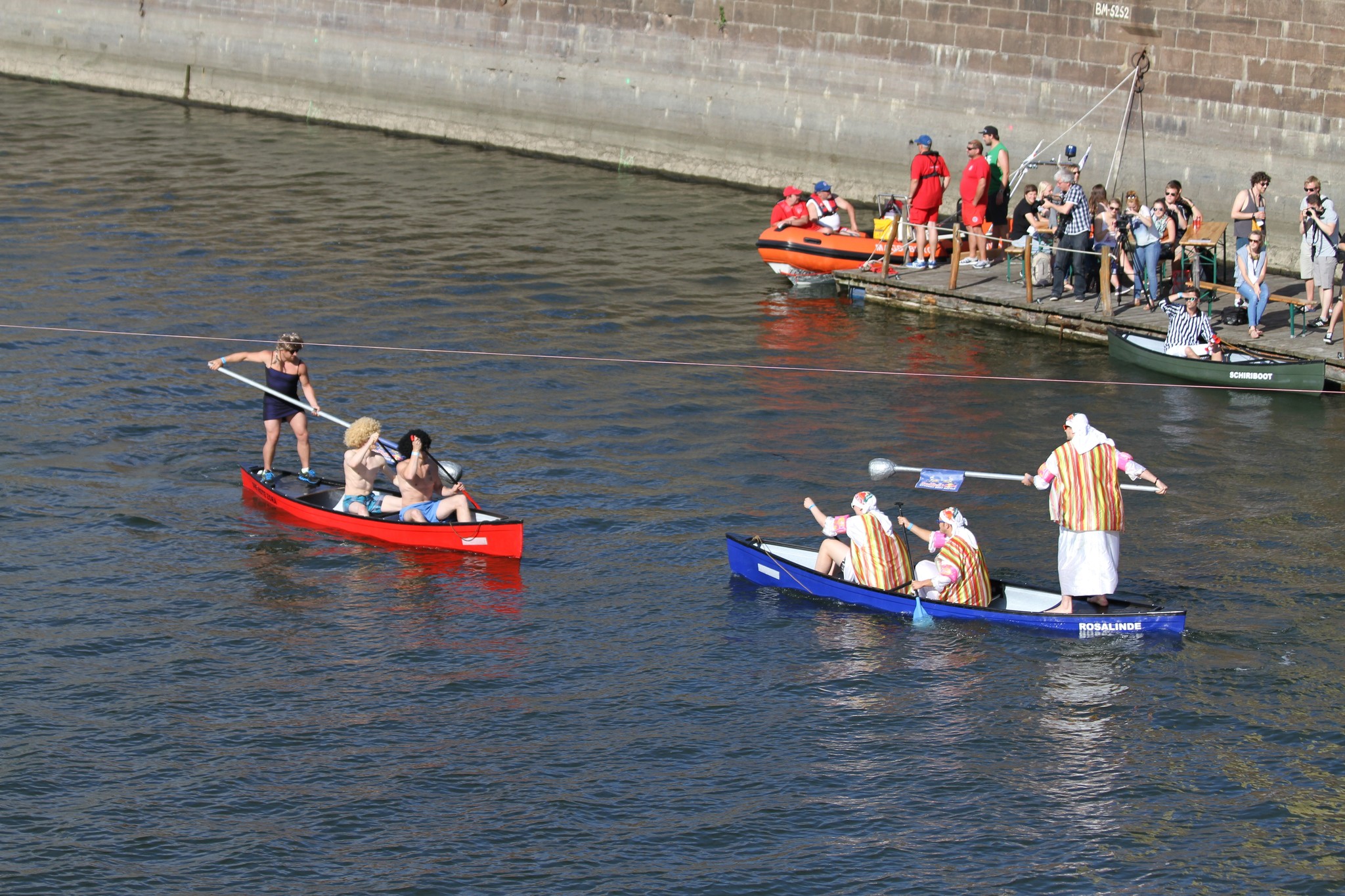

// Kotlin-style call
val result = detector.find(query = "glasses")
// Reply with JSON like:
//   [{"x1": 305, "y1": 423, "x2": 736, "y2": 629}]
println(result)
[
  {"x1": 1304, "y1": 187, "x2": 1317, "y2": 191},
  {"x1": 1063, "y1": 424, "x2": 1070, "y2": 430},
  {"x1": 421, "y1": 445, "x2": 429, "y2": 451},
  {"x1": 1248, "y1": 238, "x2": 1260, "y2": 243},
  {"x1": 851, "y1": 503, "x2": 855, "y2": 509},
  {"x1": 1258, "y1": 182, "x2": 1269, "y2": 186},
  {"x1": 1185, "y1": 298, "x2": 1195, "y2": 301},
  {"x1": 1056, "y1": 170, "x2": 1177, "y2": 210},
  {"x1": 937, "y1": 519, "x2": 945, "y2": 524},
  {"x1": 286, "y1": 349, "x2": 299, "y2": 353},
  {"x1": 967, "y1": 146, "x2": 977, "y2": 150}
]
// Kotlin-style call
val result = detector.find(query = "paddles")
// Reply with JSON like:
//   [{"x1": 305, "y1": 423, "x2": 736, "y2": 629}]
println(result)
[
  {"x1": 893, "y1": 502, "x2": 927, "y2": 619},
  {"x1": 377, "y1": 439, "x2": 398, "y2": 464},
  {"x1": 410, "y1": 434, "x2": 482, "y2": 510},
  {"x1": 833, "y1": 536, "x2": 840, "y2": 541}
]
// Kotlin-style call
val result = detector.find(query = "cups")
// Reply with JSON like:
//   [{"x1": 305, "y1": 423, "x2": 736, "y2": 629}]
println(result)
[{"x1": 1258, "y1": 207, "x2": 1264, "y2": 212}]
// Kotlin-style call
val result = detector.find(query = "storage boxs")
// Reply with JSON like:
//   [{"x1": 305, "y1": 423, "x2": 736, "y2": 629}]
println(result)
[{"x1": 873, "y1": 218, "x2": 894, "y2": 241}]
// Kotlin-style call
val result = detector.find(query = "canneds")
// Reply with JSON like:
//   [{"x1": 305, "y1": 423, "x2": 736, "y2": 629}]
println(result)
[{"x1": 1193, "y1": 216, "x2": 1200, "y2": 229}]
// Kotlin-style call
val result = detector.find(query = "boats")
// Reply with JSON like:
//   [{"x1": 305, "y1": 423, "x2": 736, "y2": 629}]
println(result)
[
  {"x1": 1102, "y1": 321, "x2": 1328, "y2": 396},
  {"x1": 756, "y1": 215, "x2": 1019, "y2": 287},
  {"x1": 240, "y1": 459, "x2": 526, "y2": 559},
  {"x1": 721, "y1": 527, "x2": 1188, "y2": 634}
]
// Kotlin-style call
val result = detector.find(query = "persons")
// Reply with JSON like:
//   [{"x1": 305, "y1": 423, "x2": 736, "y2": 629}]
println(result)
[
  {"x1": 343, "y1": 416, "x2": 402, "y2": 517},
  {"x1": 208, "y1": 332, "x2": 321, "y2": 487},
  {"x1": 395, "y1": 429, "x2": 471, "y2": 523},
  {"x1": 1323, "y1": 232, "x2": 1345, "y2": 343},
  {"x1": 803, "y1": 491, "x2": 913, "y2": 594},
  {"x1": 898, "y1": 507, "x2": 992, "y2": 607},
  {"x1": 1021, "y1": 413, "x2": 1167, "y2": 614},
  {"x1": 1158, "y1": 286, "x2": 1223, "y2": 363},
  {"x1": 770, "y1": 124, "x2": 1345, "y2": 344}
]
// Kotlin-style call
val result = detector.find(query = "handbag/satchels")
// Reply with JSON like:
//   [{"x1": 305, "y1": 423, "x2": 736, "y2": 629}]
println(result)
[
  {"x1": 1221, "y1": 306, "x2": 1247, "y2": 326},
  {"x1": 1169, "y1": 270, "x2": 1192, "y2": 294},
  {"x1": 1335, "y1": 249, "x2": 1345, "y2": 264},
  {"x1": 873, "y1": 198, "x2": 914, "y2": 240}
]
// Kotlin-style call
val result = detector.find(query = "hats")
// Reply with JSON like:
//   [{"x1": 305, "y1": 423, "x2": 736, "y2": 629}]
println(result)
[
  {"x1": 814, "y1": 181, "x2": 831, "y2": 191},
  {"x1": 979, "y1": 126, "x2": 998, "y2": 135},
  {"x1": 914, "y1": 135, "x2": 932, "y2": 146},
  {"x1": 783, "y1": 186, "x2": 802, "y2": 196}
]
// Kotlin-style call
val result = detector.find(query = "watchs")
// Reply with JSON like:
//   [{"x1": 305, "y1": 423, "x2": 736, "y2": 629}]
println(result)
[
  {"x1": 999, "y1": 189, "x2": 1004, "y2": 193},
  {"x1": 908, "y1": 195, "x2": 912, "y2": 199}
]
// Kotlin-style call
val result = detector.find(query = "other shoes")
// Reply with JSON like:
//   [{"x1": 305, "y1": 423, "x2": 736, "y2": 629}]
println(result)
[
  {"x1": 1049, "y1": 279, "x2": 1158, "y2": 310},
  {"x1": 1238, "y1": 292, "x2": 1333, "y2": 343}
]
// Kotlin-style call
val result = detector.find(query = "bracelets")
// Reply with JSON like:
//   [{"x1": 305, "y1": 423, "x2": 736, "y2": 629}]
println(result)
[
  {"x1": 1190, "y1": 203, "x2": 1195, "y2": 208},
  {"x1": 809, "y1": 504, "x2": 816, "y2": 510},
  {"x1": 411, "y1": 452, "x2": 419, "y2": 456},
  {"x1": 1178, "y1": 292, "x2": 1182, "y2": 298},
  {"x1": 221, "y1": 357, "x2": 226, "y2": 366},
  {"x1": 907, "y1": 523, "x2": 913, "y2": 530},
  {"x1": 1028, "y1": 476, "x2": 1034, "y2": 480},
  {"x1": 1139, "y1": 214, "x2": 1141, "y2": 217},
  {"x1": 1155, "y1": 477, "x2": 1158, "y2": 484},
  {"x1": 790, "y1": 220, "x2": 792, "y2": 226},
  {"x1": 1252, "y1": 212, "x2": 1255, "y2": 219}
]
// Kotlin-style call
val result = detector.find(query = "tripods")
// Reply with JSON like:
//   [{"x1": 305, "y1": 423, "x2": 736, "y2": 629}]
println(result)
[{"x1": 1094, "y1": 230, "x2": 1153, "y2": 314}]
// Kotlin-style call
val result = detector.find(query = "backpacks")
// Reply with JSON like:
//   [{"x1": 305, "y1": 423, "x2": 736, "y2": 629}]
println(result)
[{"x1": 1032, "y1": 252, "x2": 1053, "y2": 286}]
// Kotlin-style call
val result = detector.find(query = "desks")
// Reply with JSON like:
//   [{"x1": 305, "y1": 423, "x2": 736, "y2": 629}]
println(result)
[
  {"x1": 1180, "y1": 221, "x2": 1230, "y2": 319},
  {"x1": 1036, "y1": 226, "x2": 1074, "y2": 290}
]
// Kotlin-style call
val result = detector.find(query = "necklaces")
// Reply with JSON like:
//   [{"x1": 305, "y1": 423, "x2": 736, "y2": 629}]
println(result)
[{"x1": 1249, "y1": 245, "x2": 1260, "y2": 275}]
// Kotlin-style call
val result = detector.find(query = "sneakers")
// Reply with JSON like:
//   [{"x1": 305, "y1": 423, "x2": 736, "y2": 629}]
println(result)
[
  {"x1": 261, "y1": 470, "x2": 276, "y2": 485},
  {"x1": 299, "y1": 467, "x2": 320, "y2": 483},
  {"x1": 906, "y1": 258, "x2": 937, "y2": 269},
  {"x1": 959, "y1": 256, "x2": 991, "y2": 269}
]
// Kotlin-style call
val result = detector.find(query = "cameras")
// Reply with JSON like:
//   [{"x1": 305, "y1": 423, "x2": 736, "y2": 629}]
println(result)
[
  {"x1": 1040, "y1": 195, "x2": 1053, "y2": 206},
  {"x1": 1305, "y1": 207, "x2": 1323, "y2": 217}
]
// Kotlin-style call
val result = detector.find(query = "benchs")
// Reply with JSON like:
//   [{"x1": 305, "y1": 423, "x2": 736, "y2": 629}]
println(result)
[
  {"x1": 1004, "y1": 245, "x2": 1025, "y2": 285},
  {"x1": 1185, "y1": 279, "x2": 1319, "y2": 339},
  {"x1": 1145, "y1": 261, "x2": 1166, "y2": 295}
]
